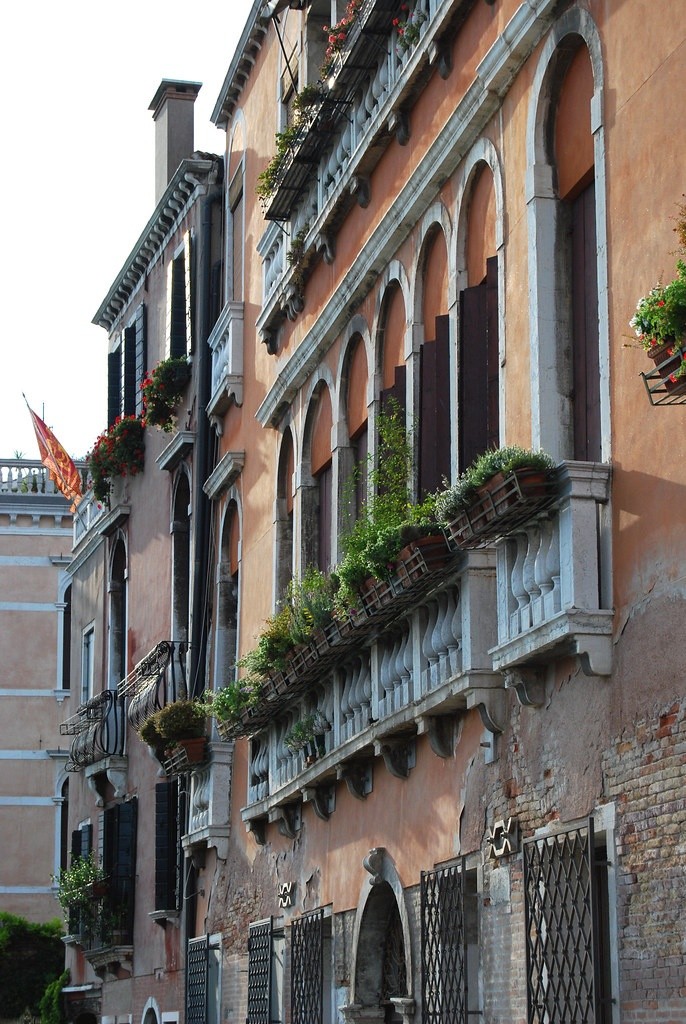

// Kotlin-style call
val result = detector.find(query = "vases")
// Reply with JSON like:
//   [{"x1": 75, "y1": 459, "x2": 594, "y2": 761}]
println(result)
[
  {"x1": 217, "y1": 468, "x2": 544, "y2": 735},
  {"x1": 647, "y1": 331, "x2": 686, "y2": 393},
  {"x1": 170, "y1": 370, "x2": 191, "y2": 389}
]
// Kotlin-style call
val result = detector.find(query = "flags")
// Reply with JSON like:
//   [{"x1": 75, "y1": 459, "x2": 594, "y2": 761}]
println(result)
[{"x1": 27, "y1": 403, "x2": 82, "y2": 513}]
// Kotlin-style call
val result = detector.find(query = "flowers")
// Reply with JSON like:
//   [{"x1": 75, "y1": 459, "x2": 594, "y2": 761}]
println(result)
[
  {"x1": 624, "y1": 260, "x2": 686, "y2": 383},
  {"x1": 194, "y1": 391, "x2": 556, "y2": 719},
  {"x1": 143, "y1": 357, "x2": 191, "y2": 434}
]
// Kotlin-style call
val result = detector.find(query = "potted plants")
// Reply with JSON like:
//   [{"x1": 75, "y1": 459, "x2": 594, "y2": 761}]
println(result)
[
  {"x1": 139, "y1": 700, "x2": 206, "y2": 767},
  {"x1": 48, "y1": 852, "x2": 108, "y2": 907}
]
